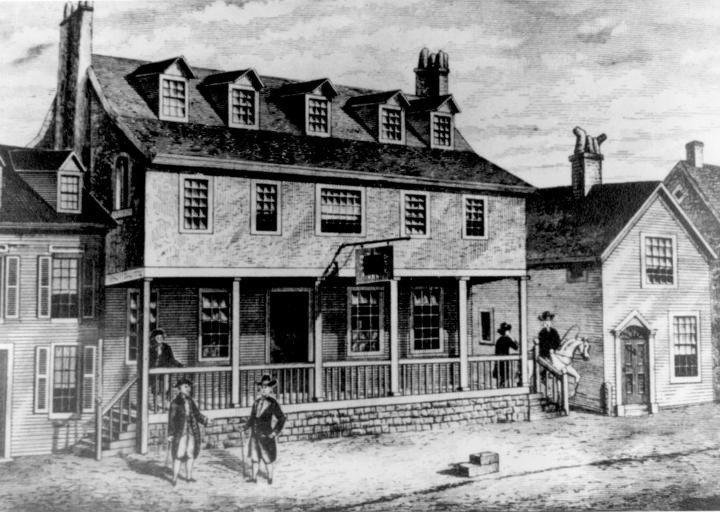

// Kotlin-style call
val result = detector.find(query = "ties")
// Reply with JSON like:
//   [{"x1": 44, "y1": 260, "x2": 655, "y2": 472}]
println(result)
[{"x1": 258, "y1": 399, "x2": 265, "y2": 417}]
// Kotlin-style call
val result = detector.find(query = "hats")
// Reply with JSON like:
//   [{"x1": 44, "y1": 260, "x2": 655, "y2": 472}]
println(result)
[
  {"x1": 171, "y1": 377, "x2": 194, "y2": 388},
  {"x1": 149, "y1": 328, "x2": 166, "y2": 343},
  {"x1": 537, "y1": 310, "x2": 556, "y2": 321},
  {"x1": 255, "y1": 374, "x2": 278, "y2": 387},
  {"x1": 496, "y1": 321, "x2": 513, "y2": 333}
]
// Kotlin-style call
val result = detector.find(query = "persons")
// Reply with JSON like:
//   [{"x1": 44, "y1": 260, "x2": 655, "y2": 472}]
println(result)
[
  {"x1": 166, "y1": 378, "x2": 207, "y2": 488},
  {"x1": 490, "y1": 319, "x2": 520, "y2": 391},
  {"x1": 534, "y1": 309, "x2": 565, "y2": 384},
  {"x1": 150, "y1": 327, "x2": 186, "y2": 413},
  {"x1": 241, "y1": 374, "x2": 288, "y2": 484}
]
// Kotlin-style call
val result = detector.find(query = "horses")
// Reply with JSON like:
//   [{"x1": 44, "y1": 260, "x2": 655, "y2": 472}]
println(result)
[{"x1": 528, "y1": 333, "x2": 590, "y2": 403}]
